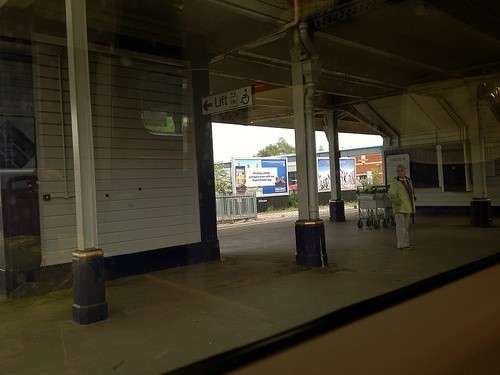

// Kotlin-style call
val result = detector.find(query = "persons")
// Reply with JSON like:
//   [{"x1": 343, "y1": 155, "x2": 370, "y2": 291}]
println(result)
[{"x1": 388, "y1": 164, "x2": 417, "y2": 252}]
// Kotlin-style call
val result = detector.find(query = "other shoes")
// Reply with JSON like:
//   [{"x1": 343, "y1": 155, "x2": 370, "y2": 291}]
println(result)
[{"x1": 402, "y1": 245, "x2": 413, "y2": 251}]
